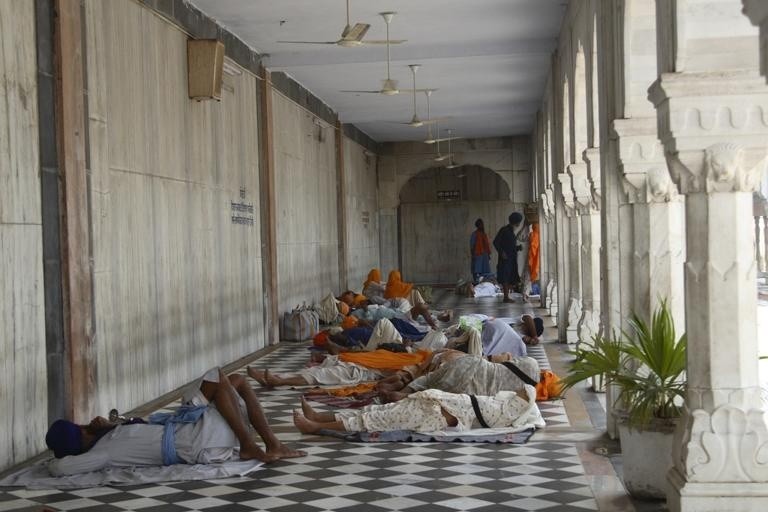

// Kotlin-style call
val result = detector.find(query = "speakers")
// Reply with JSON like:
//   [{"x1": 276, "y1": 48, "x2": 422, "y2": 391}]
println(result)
[{"x1": 188, "y1": 38, "x2": 225, "y2": 102}]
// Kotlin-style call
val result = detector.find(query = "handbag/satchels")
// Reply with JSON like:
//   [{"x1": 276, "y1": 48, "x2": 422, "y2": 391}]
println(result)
[{"x1": 283, "y1": 304, "x2": 320, "y2": 342}]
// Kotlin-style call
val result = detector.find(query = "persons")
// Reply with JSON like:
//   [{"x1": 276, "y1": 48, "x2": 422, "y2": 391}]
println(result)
[
  {"x1": 45, "y1": 366, "x2": 308, "y2": 478},
  {"x1": 493, "y1": 212, "x2": 523, "y2": 302},
  {"x1": 246, "y1": 269, "x2": 563, "y2": 435},
  {"x1": 470, "y1": 218, "x2": 491, "y2": 282},
  {"x1": 516, "y1": 215, "x2": 541, "y2": 295}
]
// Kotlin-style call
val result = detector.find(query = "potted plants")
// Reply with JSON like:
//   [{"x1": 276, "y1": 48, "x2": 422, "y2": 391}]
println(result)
[{"x1": 548, "y1": 290, "x2": 689, "y2": 500}]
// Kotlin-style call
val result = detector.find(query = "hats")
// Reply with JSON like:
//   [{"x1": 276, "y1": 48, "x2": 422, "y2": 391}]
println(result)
[
  {"x1": 44, "y1": 419, "x2": 83, "y2": 456},
  {"x1": 341, "y1": 315, "x2": 359, "y2": 330},
  {"x1": 337, "y1": 301, "x2": 351, "y2": 314},
  {"x1": 353, "y1": 294, "x2": 368, "y2": 306},
  {"x1": 313, "y1": 332, "x2": 329, "y2": 346},
  {"x1": 534, "y1": 317, "x2": 544, "y2": 337},
  {"x1": 509, "y1": 212, "x2": 523, "y2": 224}
]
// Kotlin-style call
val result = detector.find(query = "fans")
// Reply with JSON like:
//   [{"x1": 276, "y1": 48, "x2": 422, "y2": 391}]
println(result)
[
  {"x1": 340, "y1": 12, "x2": 464, "y2": 170},
  {"x1": 276, "y1": 0, "x2": 407, "y2": 51}
]
